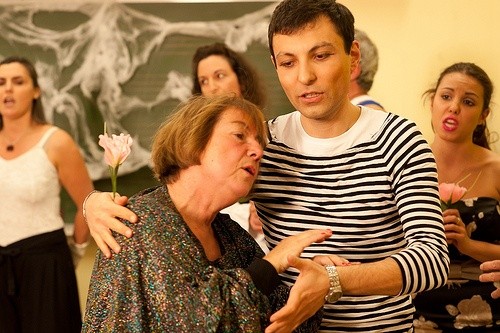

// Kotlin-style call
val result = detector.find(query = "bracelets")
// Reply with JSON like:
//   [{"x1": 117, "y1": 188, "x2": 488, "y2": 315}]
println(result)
[
  {"x1": 71, "y1": 240, "x2": 90, "y2": 249},
  {"x1": 83, "y1": 190, "x2": 100, "y2": 223}
]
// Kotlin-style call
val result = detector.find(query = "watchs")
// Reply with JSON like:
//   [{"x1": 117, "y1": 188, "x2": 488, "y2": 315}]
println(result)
[{"x1": 325, "y1": 266, "x2": 342, "y2": 303}]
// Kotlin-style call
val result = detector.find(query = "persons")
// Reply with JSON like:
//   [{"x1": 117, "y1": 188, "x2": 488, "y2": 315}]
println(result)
[
  {"x1": 84, "y1": 94, "x2": 350, "y2": 333},
  {"x1": 0, "y1": 55, "x2": 97, "y2": 333},
  {"x1": 191, "y1": 42, "x2": 269, "y2": 256},
  {"x1": 350, "y1": 30, "x2": 388, "y2": 114},
  {"x1": 83, "y1": 0, "x2": 450, "y2": 333},
  {"x1": 411, "y1": 63, "x2": 500, "y2": 333}
]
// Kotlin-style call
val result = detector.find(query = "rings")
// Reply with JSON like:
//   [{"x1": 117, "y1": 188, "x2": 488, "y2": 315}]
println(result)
[{"x1": 457, "y1": 218, "x2": 460, "y2": 224}]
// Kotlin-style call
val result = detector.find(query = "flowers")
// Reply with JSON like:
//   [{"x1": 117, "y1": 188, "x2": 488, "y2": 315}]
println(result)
[
  {"x1": 439, "y1": 172, "x2": 481, "y2": 209},
  {"x1": 99, "y1": 132, "x2": 133, "y2": 198}
]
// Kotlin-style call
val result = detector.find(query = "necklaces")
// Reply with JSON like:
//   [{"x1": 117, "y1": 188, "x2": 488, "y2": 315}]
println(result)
[{"x1": 4, "y1": 126, "x2": 32, "y2": 152}]
